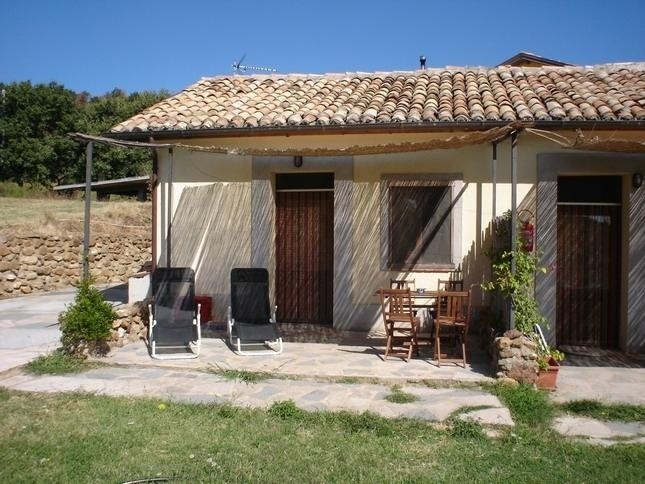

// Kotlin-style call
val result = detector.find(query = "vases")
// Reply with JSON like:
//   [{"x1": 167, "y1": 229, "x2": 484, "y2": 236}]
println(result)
[{"x1": 536, "y1": 355, "x2": 560, "y2": 391}]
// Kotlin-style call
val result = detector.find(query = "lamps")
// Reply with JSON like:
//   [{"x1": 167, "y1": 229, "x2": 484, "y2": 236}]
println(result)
[
  {"x1": 293, "y1": 155, "x2": 304, "y2": 168},
  {"x1": 631, "y1": 171, "x2": 643, "y2": 187}
]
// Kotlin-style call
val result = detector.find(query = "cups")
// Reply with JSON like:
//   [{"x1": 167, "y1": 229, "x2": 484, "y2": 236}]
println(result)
[{"x1": 417, "y1": 288, "x2": 423, "y2": 293}]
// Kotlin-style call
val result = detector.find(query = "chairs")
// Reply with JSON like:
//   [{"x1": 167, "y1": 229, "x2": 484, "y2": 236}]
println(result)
[
  {"x1": 388, "y1": 277, "x2": 418, "y2": 354},
  {"x1": 226, "y1": 266, "x2": 284, "y2": 356},
  {"x1": 378, "y1": 287, "x2": 421, "y2": 363},
  {"x1": 428, "y1": 277, "x2": 465, "y2": 354},
  {"x1": 430, "y1": 287, "x2": 474, "y2": 369},
  {"x1": 146, "y1": 267, "x2": 204, "y2": 361}
]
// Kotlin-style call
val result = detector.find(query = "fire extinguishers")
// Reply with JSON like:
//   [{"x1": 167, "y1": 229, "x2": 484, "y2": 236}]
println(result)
[{"x1": 517, "y1": 208, "x2": 534, "y2": 252}]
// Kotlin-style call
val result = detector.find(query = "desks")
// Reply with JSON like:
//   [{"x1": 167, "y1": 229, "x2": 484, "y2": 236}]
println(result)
[{"x1": 372, "y1": 288, "x2": 466, "y2": 357}]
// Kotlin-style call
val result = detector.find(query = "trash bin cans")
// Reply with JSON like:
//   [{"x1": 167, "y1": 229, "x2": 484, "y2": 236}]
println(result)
[
  {"x1": 136, "y1": 188, "x2": 147, "y2": 201},
  {"x1": 97, "y1": 187, "x2": 110, "y2": 201}
]
